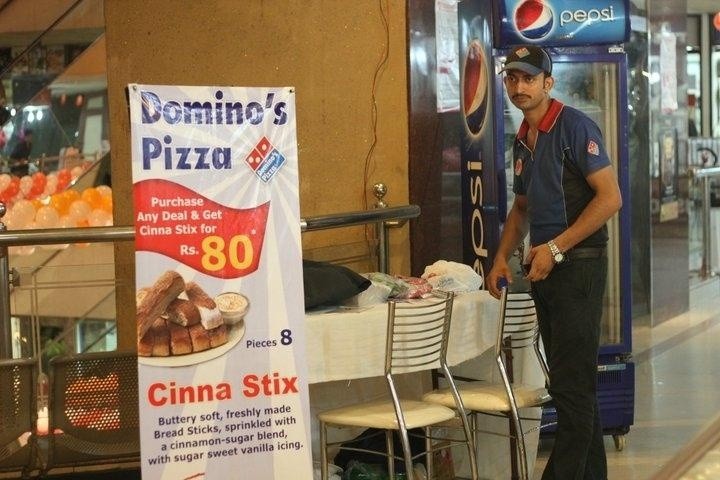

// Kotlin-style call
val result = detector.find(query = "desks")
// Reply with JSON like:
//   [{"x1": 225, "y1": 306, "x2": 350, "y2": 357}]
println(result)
[{"x1": 303, "y1": 288, "x2": 519, "y2": 480}]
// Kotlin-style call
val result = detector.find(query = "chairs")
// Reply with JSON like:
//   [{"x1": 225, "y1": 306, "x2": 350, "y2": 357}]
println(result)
[
  {"x1": 46, "y1": 350, "x2": 142, "y2": 479},
  {"x1": 422, "y1": 280, "x2": 554, "y2": 480},
  {"x1": 317, "y1": 290, "x2": 478, "y2": 479},
  {"x1": 0, "y1": 357, "x2": 46, "y2": 480}
]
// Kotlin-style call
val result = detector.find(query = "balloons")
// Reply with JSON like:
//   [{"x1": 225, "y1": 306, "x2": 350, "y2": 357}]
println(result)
[
  {"x1": 18, "y1": 373, "x2": 123, "y2": 451},
  {"x1": 0, "y1": 167, "x2": 115, "y2": 256}
]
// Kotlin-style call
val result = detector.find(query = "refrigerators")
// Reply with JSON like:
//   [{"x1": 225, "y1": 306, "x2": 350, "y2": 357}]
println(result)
[{"x1": 458, "y1": 0, "x2": 636, "y2": 451}]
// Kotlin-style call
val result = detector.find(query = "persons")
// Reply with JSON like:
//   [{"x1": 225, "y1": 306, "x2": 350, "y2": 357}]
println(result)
[
  {"x1": 7, "y1": 127, "x2": 34, "y2": 178},
  {"x1": 485, "y1": 42, "x2": 625, "y2": 480}
]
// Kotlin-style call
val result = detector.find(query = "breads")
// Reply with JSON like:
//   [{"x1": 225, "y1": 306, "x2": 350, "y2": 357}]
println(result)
[{"x1": 137, "y1": 271, "x2": 229, "y2": 358}]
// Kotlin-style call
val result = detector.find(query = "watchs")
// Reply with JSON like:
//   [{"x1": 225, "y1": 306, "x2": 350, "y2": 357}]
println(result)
[{"x1": 548, "y1": 240, "x2": 565, "y2": 265}]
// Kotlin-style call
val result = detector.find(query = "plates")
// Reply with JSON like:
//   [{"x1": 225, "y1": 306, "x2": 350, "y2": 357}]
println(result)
[{"x1": 137, "y1": 322, "x2": 246, "y2": 368}]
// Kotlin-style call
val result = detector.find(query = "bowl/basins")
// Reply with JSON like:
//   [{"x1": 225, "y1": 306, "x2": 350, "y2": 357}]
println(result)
[{"x1": 211, "y1": 293, "x2": 250, "y2": 329}]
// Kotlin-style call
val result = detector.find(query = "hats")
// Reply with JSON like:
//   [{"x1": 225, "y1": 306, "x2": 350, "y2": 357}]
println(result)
[{"x1": 498, "y1": 45, "x2": 552, "y2": 76}]
